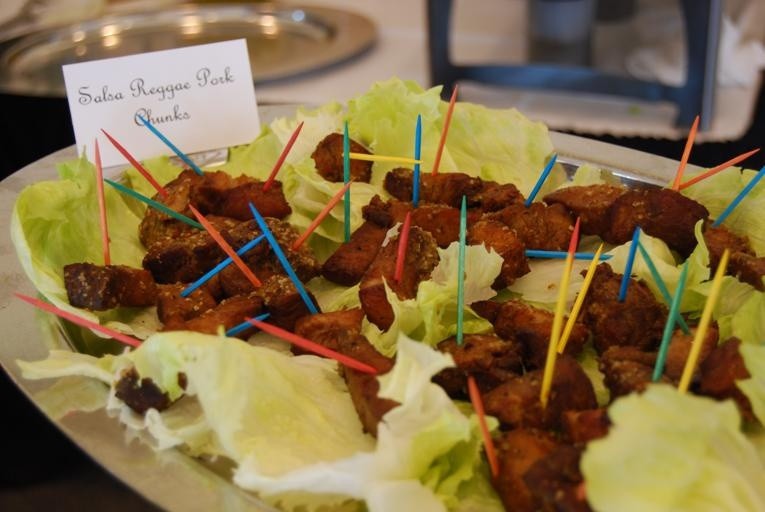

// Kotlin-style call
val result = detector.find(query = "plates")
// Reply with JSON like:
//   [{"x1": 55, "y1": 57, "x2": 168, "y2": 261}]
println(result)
[{"x1": 0, "y1": 103, "x2": 707, "y2": 512}]
